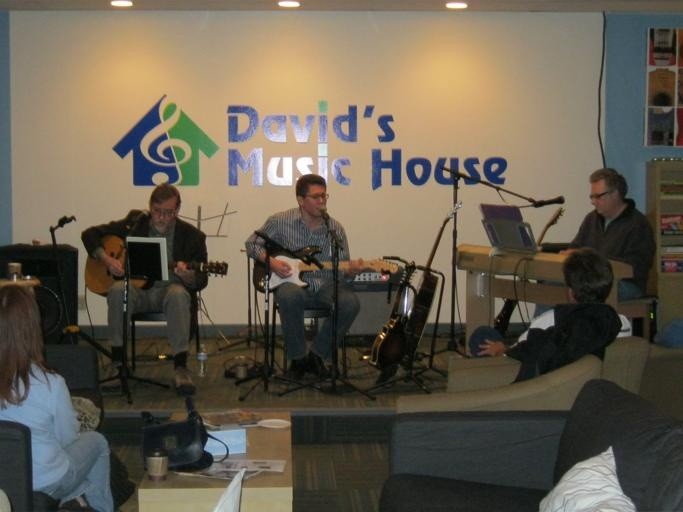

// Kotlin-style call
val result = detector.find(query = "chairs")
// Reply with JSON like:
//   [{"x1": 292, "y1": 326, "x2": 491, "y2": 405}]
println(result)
[{"x1": 0, "y1": 420, "x2": 55, "y2": 512}]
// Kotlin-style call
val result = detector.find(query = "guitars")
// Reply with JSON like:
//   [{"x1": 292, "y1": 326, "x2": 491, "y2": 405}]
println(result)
[
  {"x1": 399, "y1": 199, "x2": 464, "y2": 373},
  {"x1": 252, "y1": 248, "x2": 400, "y2": 294},
  {"x1": 368, "y1": 260, "x2": 418, "y2": 376},
  {"x1": 84, "y1": 234, "x2": 229, "y2": 296},
  {"x1": 495, "y1": 206, "x2": 565, "y2": 337}
]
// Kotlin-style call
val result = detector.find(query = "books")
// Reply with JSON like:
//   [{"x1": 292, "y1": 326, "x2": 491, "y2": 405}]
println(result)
[
  {"x1": 203, "y1": 407, "x2": 262, "y2": 431},
  {"x1": 662, "y1": 246, "x2": 683, "y2": 272},
  {"x1": 659, "y1": 214, "x2": 683, "y2": 237}
]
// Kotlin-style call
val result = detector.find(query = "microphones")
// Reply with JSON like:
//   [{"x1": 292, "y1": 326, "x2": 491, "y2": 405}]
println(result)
[
  {"x1": 534, "y1": 195, "x2": 564, "y2": 207},
  {"x1": 133, "y1": 210, "x2": 147, "y2": 232},
  {"x1": 320, "y1": 206, "x2": 329, "y2": 224}
]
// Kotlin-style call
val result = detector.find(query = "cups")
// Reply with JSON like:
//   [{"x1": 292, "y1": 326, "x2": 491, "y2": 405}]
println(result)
[
  {"x1": 8, "y1": 263, "x2": 21, "y2": 281},
  {"x1": 145, "y1": 449, "x2": 169, "y2": 481}
]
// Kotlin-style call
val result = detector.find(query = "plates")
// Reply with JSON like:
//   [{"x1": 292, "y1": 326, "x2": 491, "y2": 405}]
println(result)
[{"x1": 258, "y1": 419, "x2": 291, "y2": 429}]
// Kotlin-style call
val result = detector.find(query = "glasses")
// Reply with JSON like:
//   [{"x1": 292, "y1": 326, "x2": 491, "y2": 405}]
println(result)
[
  {"x1": 590, "y1": 192, "x2": 609, "y2": 200},
  {"x1": 150, "y1": 207, "x2": 180, "y2": 218},
  {"x1": 305, "y1": 193, "x2": 330, "y2": 200}
]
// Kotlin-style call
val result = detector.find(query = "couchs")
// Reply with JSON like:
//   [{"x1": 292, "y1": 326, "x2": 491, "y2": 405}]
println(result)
[
  {"x1": 397, "y1": 335, "x2": 682, "y2": 412},
  {"x1": 377, "y1": 378, "x2": 681, "y2": 512}
]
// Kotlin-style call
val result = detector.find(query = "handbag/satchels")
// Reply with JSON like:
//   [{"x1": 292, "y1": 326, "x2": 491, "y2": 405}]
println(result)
[{"x1": 141, "y1": 411, "x2": 229, "y2": 472}]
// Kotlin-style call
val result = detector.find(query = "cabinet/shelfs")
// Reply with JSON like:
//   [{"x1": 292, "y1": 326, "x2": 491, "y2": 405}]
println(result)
[{"x1": 646, "y1": 159, "x2": 683, "y2": 333}]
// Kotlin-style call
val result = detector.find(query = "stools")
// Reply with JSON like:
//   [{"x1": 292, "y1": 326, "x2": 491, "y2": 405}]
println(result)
[
  {"x1": 43, "y1": 343, "x2": 98, "y2": 391},
  {"x1": 618, "y1": 302, "x2": 651, "y2": 344},
  {"x1": 130, "y1": 311, "x2": 200, "y2": 373},
  {"x1": 276, "y1": 306, "x2": 348, "y2": 380}
]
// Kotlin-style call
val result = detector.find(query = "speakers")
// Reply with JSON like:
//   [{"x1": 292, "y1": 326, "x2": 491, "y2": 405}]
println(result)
[{"x1": 0, "y1": 244, "x2": 78, "y2": 344}]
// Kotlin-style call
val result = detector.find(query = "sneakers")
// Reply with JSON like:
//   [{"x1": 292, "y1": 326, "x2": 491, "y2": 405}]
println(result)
[
  {"x1": 285, "y1": 362, "x2": 306, "y2": 380},
  {"x1": 172, "y1": 371, "x2": 195, "y2": 395},
  {"x1": 301, "y1": 350, "x2": 328, "y2": 379}
]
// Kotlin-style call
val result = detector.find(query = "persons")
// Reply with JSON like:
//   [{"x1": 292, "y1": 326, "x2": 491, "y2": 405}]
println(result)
[
  {"x1": 0, "y1": 284, "x2": 114, "y2": 511},
  {"x1": 533, "y1": 168, "x2": 657, "y2": 319},
  {"x1": 468, "y1": 250, "x2": 633, "y2": 385},
  {"x1": 81, "y1": 184, "x2": 208, "y2": 395},
  {"x1": 245, "y1": 175, "x2": 364, "y2": 381}
]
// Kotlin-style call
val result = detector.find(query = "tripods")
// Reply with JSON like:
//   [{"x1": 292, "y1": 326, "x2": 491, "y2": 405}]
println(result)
[
  {"x1": 367, "y1": 281, "x2": 432, "y2": 394},
  {"x1": 235, "y1": 229, "x2": 323, "y2": 400},
  {"x1": 217, "y1": 252, "x2": 283, "y2": 353},
  {"x1": 415, "y1": 265, "x2": 446, "y2": 379},
  {"x1": 99, "y1": 232, "x2": 171, "y2": 405},
  {"x1": 422, "y1": 165, "x2": 533, "y2": 359},
  {"x1": 277, "y1": 220, "x2": 376, "y2": 401}
]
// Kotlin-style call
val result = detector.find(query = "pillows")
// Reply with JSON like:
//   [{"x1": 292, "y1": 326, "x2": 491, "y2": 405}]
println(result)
[{"x1": 538, "y1": 446, "x2": 639, "y2": 512}]
